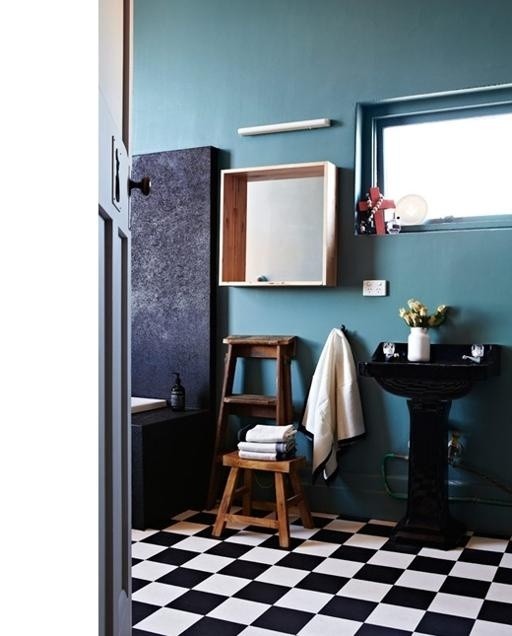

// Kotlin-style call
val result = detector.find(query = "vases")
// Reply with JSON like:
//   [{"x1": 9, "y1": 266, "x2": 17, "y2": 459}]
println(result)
[{"x1": 407, "y1": 326, "x2": 431, "y2": 362}]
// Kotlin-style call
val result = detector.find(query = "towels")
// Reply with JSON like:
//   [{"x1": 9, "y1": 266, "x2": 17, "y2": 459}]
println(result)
[
  {"x1": 238, "y1": 450, "x2": 296, "y2": 461},
  {"x1": 297, "y1": 327, "x2": 366, "y2": 487},
  {"x1": 245, "y1": 424, "x2": 297, "y2": 443},
  {"x1": 237, "y1": 441, "x2": 295, "y2": 453}
]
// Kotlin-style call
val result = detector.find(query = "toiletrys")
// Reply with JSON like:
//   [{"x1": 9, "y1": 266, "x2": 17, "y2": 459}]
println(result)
[{"x1": 169, "y1": 371, "x2": 186, "y2": 412}]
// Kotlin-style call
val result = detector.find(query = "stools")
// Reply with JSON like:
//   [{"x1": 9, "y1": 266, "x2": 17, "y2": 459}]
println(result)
[{"x1": 211, "y1": 452, "x2": 315, "y2": 549}]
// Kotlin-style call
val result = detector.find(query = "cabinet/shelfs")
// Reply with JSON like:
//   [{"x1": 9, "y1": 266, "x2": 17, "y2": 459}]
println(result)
[{"x1": 216, "y1": 161, "x2": 339, "y2": 288}]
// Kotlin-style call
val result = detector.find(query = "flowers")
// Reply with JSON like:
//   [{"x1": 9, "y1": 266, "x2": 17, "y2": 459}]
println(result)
[{"x1": 398, "y1": 298, "x2": 448, "y2": 328}]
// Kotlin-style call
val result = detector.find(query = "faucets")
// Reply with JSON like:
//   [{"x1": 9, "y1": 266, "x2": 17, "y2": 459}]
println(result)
[
  {"x1": 462, "y1": 344, "x2": 484, "y2": 363},
  {"x1": 383, "y1": 343, "x2": 399, "y2": 361}
]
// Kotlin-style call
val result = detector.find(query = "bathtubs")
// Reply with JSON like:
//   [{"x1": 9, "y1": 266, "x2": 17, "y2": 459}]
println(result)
[{"x1": 131, "y1": 396, "x2": 167, "y2": 414}]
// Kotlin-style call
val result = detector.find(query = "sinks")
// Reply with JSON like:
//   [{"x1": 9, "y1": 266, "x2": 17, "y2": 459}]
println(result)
[{"x1": 358, "y1": 361, "x2": 489, "y2": 399}]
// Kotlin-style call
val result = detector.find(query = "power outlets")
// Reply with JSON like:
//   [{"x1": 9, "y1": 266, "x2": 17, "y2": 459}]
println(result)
[{"x1": 362, "y1": 279, "x2": 387, "y2": 297}]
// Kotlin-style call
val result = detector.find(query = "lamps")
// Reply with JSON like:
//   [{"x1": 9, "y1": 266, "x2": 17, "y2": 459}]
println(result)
[{"x1": 238, "y1": 118, "x2": 332, "y2": 137}]
